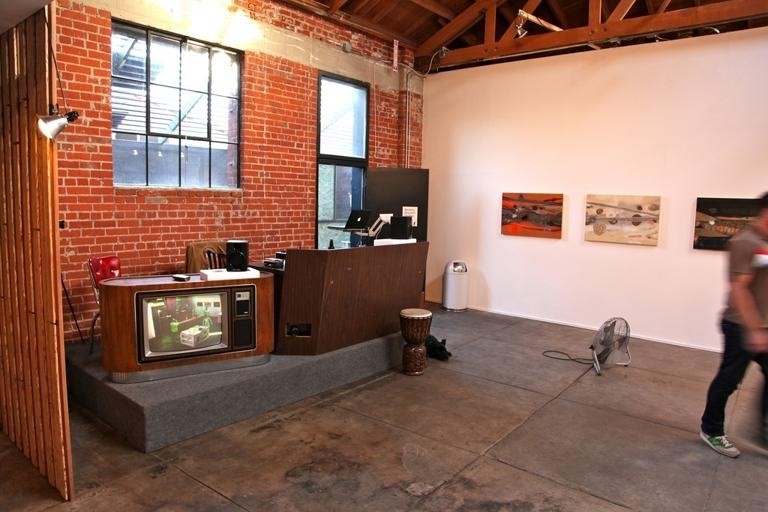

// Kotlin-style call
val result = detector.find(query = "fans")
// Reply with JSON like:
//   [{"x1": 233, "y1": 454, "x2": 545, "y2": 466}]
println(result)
[{"x1": 592, "y1": 316, "x2": 632, "y2": 376}]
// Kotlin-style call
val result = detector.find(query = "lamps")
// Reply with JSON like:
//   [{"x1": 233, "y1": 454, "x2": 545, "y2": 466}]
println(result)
[{"x1": 37, "y1": 111, "x2": 78, "y2": 142}]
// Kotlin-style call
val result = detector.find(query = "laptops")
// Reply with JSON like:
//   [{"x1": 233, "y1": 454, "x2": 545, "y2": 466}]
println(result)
[{"x1": 328, "y1": 209, "x2": 372, "y2": 229}]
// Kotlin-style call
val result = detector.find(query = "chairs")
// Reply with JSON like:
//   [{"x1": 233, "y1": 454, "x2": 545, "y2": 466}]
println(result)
[
  {"x1": 186, "y1": 239, "x2": 226, "y2": 272},
  {"x1": 87, "y1": 256, "x2": 121, "y2": 355}
]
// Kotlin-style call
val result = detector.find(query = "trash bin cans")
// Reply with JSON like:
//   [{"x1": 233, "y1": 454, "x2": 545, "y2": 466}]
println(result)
[{"x1": 441, "y1": 260, "x2": 468, "y2": 312}]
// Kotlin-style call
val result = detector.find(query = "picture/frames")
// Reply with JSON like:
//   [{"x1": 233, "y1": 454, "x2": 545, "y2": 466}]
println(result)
[
  {"x1": 500, "y1": 192, "x2": 563, "y2": 240},
  {"x1": 693, "y1": 197, "x2": 760, "y2": 251},
  {"x1": 584, "y1": 194, "x2": 661, "y2": 246}
]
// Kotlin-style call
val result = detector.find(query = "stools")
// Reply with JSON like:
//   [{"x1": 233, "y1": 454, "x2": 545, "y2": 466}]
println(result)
[{"x1": 400, "y1": 309, "x2": 433, "y2": 377}]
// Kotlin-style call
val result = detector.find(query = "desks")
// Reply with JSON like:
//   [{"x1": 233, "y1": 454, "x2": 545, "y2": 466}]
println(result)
[
  {"x1": 248, "y1": 241, "x2": 429, "y2": 356},
  {"x1": 99, "y1": 270, "x2": 275, "y2": 383}
]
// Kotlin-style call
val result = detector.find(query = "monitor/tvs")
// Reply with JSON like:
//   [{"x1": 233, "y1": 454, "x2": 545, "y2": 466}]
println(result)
[
  {"x1": 133, "y1": 284, "x2": 257, "y2": 365},
  {"x1": 382, "y1": 215, "x2": 412, "y2": 239}
]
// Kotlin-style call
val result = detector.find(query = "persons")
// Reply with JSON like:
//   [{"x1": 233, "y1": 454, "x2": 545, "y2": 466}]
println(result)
[{"x1": 700, "y1": 190, "x2": 768, "y2": 458}]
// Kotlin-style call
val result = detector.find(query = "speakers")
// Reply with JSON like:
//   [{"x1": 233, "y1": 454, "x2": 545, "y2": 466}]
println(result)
[{"x1": 226, "y1": 239, "x2": 249, "y2": 271}]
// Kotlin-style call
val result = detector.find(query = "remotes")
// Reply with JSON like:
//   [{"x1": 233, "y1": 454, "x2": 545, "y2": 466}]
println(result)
[{"x1": 172, "y1": 274, "x2": 190, "y2": 281}]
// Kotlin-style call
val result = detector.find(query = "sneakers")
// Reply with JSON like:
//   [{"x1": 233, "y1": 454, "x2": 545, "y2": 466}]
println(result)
[
  {"x1": 743, "y1": 437, "x2": 768, "y2": 460},
  {"x1": 699, "y1": 428, "x2": 742, "y2": 461}
]
